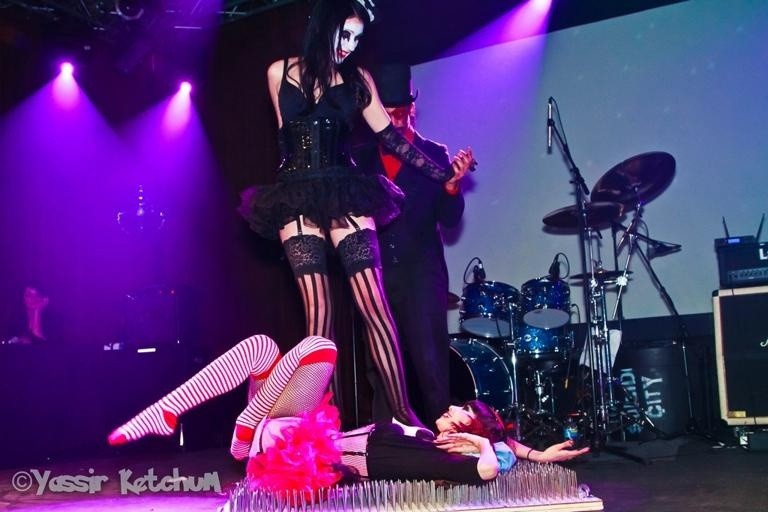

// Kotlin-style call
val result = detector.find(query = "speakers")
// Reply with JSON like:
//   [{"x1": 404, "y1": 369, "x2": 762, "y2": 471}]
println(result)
[{"x1": 711, "y1": 284, "x2": 768, "y2": 427}]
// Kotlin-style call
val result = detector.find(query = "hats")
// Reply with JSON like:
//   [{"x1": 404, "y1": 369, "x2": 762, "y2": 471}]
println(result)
[{"x1": 368, "y1": 60, "x2": 418, "y2": 109}]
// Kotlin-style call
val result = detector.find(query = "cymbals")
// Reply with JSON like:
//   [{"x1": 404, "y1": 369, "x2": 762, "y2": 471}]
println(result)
[
  {"x1": 543, "y1": 202, "x2": 621, "y2": 227},
  {"x1": 591, "y1": 153, "x2": 675, "y2": 212},
  {"x1": 571, "y1": 271, "x2": 632, "y2": 279}
]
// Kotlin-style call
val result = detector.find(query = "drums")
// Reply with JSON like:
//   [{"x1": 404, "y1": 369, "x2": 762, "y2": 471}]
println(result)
[
  {"x1": 459, "y1": 281, "x2": 521, "y2": 339},
  {"x1": 521, "y1": 278, "x2": 571, "y2": 330},
  {"x1": 448, "y1": 338, "x2": 514, "y2": 423},
  {"x1": 514, "y1": 322, "x2": 573, "y2": 359}
]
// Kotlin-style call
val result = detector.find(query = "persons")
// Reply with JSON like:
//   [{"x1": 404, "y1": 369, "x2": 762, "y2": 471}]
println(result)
[
  {"x1": 247, "y1": 0, "x2": 481, "y2": 442},
  {"x1": 8, "y1": 281, "x2": 76, "y2": 346},
  {"x1": 373, "y1": 103, "x2": 475, "y2": 434},
  {"x1": 106, "y1": 333, "x2": 593, "y2": 506}
]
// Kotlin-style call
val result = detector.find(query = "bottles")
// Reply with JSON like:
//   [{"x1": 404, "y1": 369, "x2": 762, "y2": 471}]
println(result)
[{"x1": 561, "y1": 414, "x2": 581, "y2": 450}]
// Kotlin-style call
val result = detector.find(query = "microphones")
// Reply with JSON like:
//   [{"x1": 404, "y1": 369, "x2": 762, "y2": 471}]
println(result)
[
  {"x1": 478, "y1": 260, "x2": 486, "y2": 279},
  {"x1": 547, "y1": 103, "x2": 556, "y2": 154}
]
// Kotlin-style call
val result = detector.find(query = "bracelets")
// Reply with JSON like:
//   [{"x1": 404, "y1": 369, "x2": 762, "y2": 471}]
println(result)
[{"x1": 527, "y1": 448, "x2": 533, "y2": 460}]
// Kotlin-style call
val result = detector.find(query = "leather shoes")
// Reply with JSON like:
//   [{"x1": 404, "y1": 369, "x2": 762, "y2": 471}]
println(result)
[{"x1": 392, "y1": 417, "x2": 434, "y2": 438}]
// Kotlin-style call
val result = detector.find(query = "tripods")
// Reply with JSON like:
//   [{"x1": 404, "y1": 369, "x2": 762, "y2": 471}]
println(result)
[{"x1": 488, "y1": 124, "x2": 727, "y2": 467}]
